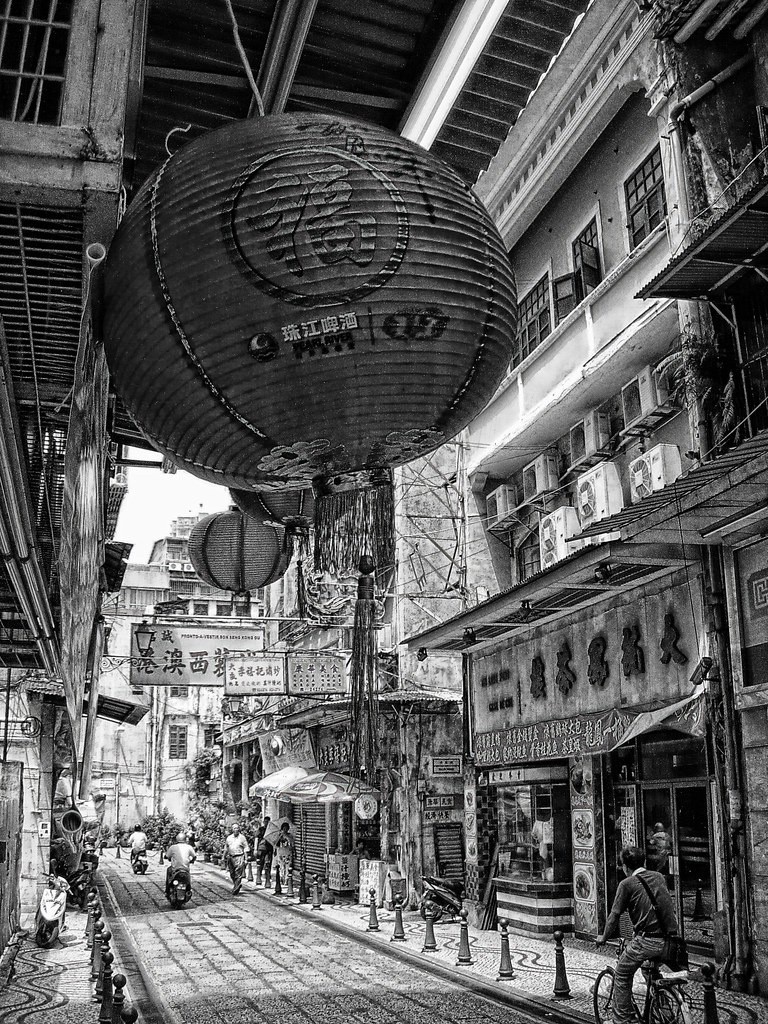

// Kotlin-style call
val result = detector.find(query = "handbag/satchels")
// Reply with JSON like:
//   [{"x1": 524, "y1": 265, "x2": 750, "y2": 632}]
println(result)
[{"x1": 668, "y1": 937, "x2": 688, "y2": 970}]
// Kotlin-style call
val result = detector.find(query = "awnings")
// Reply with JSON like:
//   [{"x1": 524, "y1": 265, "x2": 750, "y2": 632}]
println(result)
[
  {"x1": 97, "y1": 695, "x2": 150, "y2": 726},
  {"x1": 565, "y1": 428, "x2": 768, "y2": 543},
  {"x1": 634, "y1": 174, "x2": 768, "y2": 300},
  {"x1": 104, "y1": 539, "x2": 133, "y2": 592}
]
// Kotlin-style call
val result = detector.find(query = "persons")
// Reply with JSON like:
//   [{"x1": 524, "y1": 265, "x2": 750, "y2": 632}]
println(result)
[
  {"x1": 220, "y1": 823, "x2": 250, "y2": 895},
  {"x1": 274, "y1": 822, "x2": 296, "y2": 886},
  {"x1": 127, "y1": 824, "x2": 148, "y2": 866},
  {"x1": 254, "y1": 816, "x2": 277, "y2": 888},
  {"x1": 596, "y1": 846, "x2": 676, "y2": 1024},
  {"x1": 164, "y1": 832, "x2": 197, "y2": 897},
  {"x1": 647, "y1": 822, "x2": 674, "y2": 892}
]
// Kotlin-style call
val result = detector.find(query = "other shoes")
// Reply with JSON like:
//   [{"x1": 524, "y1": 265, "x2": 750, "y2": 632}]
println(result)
[
  {"x1": 603, "y1": 1019, "x2": 613, "y2": 1024},
  {"x1": 232, "y1": 882, "x2": 242, "y2": 895}
]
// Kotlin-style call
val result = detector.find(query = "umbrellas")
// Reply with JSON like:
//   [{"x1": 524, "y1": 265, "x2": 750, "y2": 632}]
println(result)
[
  {"x1": 249, "y1": 765, "x2": 326, "y2": 817},
  {"x1": 263, "y1": 816, "x2": 298, "y2": 847},
  {"x1": 274, "y1": 771, "x2": 381, "y2": 851}
]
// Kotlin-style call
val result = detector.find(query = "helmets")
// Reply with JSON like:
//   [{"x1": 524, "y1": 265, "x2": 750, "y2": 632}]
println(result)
[
  {"x1": 135, "y1": 824, "x2": 141, "y2": 831},
  {"x1": 176, "y1": 833, "x2": 186, "y2": 842}
]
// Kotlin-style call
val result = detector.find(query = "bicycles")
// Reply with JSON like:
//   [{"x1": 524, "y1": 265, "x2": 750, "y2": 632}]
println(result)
[{"x1": 593, "y1": 936, "x2": 695, "y2": 1024}]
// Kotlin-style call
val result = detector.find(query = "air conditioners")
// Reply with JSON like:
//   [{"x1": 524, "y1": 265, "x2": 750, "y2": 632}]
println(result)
[
  {"x1": 485, "y1": 362, "x2": 682, "y2": 573},
  {"x1": 168, "y1": 562, "x2": 195, "y2": 572}
]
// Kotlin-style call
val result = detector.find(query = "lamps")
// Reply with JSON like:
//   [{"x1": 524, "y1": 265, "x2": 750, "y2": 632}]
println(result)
[
  {"x1": 417, "y1": 647, "x2": 428, "y2": 661},
  {"x1": 519, "y1": 599, "x2": 533, "y2": 621},
  {"x1": 226, "y1": 695, "x2": 244, "y2": 715},
  {"x1": 101, "y1": 620, "x2": 156, "y2": 673},
  {"x1": 594, "y1": 562, "x2": 612, "y2": 583},
  {"x1": 463, "y1": 627, "x2": 477, "y2": 645}
]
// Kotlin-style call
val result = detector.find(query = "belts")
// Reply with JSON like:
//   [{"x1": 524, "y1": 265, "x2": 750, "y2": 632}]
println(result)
[
  {"x1": 228, "y1": 853, "x2": 244, "y2": 858},
  {"x1": 638, "y1": 932, "x2": 669, "y2": 938}
]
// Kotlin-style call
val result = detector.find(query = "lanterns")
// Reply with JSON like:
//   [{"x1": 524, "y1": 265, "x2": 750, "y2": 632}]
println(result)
[
  {"x1": 181, "y1": 486, "x2": 379, "y2": 634},
  {"x1": 92, "y1": 110, "x2": 517, "y2": 566}
]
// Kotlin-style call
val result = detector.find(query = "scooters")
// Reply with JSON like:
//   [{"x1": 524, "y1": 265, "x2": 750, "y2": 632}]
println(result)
[
  {"x1": 33, "y1": 857, "x2": 75, "y2": 949},
  {"x1": 163, "y1": 854, "x2": 195, "y2": 910},
  {"x1": 417, "y1": 875, "x2": 468, "y2": 923},
  {"x1": 128, "y1": 843, "x2": 149, "y2": 874}
]
